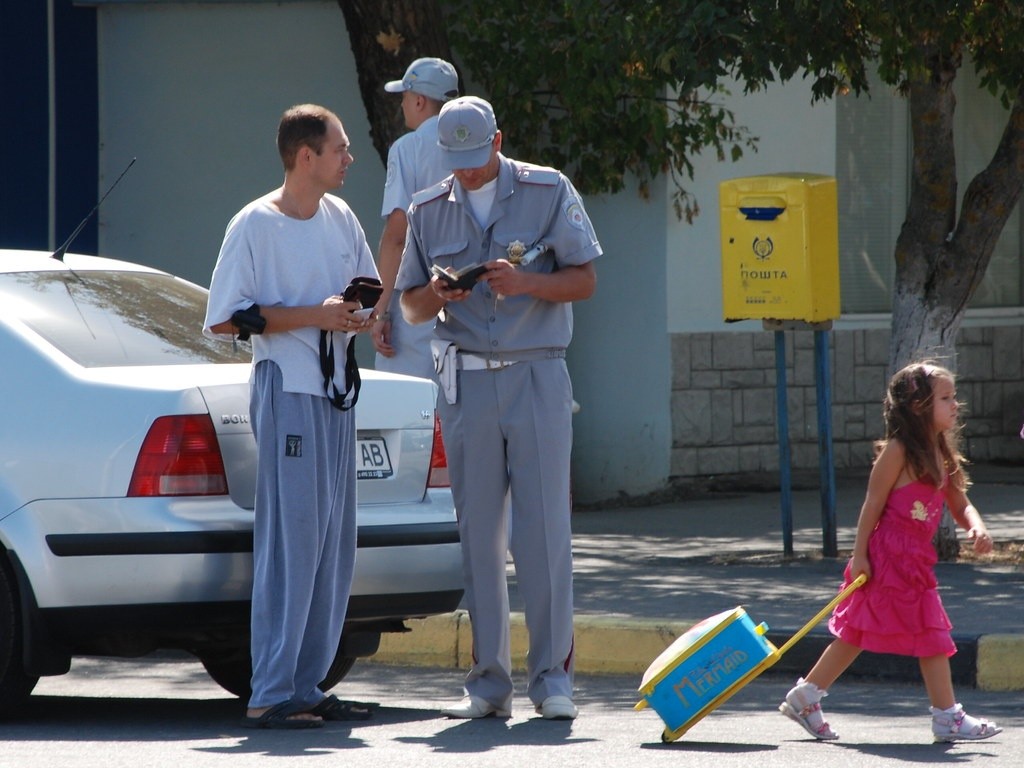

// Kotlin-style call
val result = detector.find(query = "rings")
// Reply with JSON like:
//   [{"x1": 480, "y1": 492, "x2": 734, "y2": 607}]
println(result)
[
  {"x1": 347, "y1": 320, "x2": 351, "y2": 328},
  {"x1": 491, "y1": 269, "x2": 497, "y2": 280}
]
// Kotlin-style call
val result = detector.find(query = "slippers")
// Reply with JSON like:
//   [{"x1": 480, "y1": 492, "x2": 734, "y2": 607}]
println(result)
[{"x1": 240, "y1": 693, "x2": 377, "y2": 728}]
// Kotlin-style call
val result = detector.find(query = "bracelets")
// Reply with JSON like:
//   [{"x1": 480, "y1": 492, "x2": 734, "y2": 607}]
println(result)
[{"x1": 376, "y1": 311, "x2": 391, "y2": 322}]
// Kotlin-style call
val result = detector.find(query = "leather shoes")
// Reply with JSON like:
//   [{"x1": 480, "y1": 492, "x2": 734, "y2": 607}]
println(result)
[{"x1": 441, "y1": 695, "x2": 578, "y2": 719}]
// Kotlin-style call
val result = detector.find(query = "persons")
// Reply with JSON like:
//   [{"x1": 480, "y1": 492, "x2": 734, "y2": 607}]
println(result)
[
  {"x1": 393, "y1": 93, "x2": 605, "y2": 719},
  {"x1": 779, "y1": 360, "x2": 1003, "y2": 744},
  {"x1": 371, "y1": 59, "x2": 459, "y2": 381},
  {"x1": 201, "y1": 101, "x2": 385, "y2": 730}
]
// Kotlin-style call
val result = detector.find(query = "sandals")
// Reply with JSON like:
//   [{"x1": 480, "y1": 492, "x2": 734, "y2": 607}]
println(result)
[
  {"x1": 931, "y1": 710, "x2": 1003, "y2": 743},
  {"x1": 779, "y1": 686, "x2": 839, "y2": 740}
]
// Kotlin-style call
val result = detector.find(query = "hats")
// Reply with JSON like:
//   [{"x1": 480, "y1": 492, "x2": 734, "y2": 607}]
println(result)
[
  {"x1": 384, "y1": 56, "x2": 459, "y2": 101},
  {"x1": 436, "y1": 95, "x2": 497, "y2": 170}
]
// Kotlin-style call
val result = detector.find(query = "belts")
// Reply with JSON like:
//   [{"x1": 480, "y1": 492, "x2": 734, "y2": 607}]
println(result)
[{"x1": 457, "y1": 355, "x2": 520, "y2": 369}]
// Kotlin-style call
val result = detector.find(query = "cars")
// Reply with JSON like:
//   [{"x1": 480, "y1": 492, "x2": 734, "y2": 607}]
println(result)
[{"x1": 1, "y1": 153, "x2": 466, "y2": 718}]
